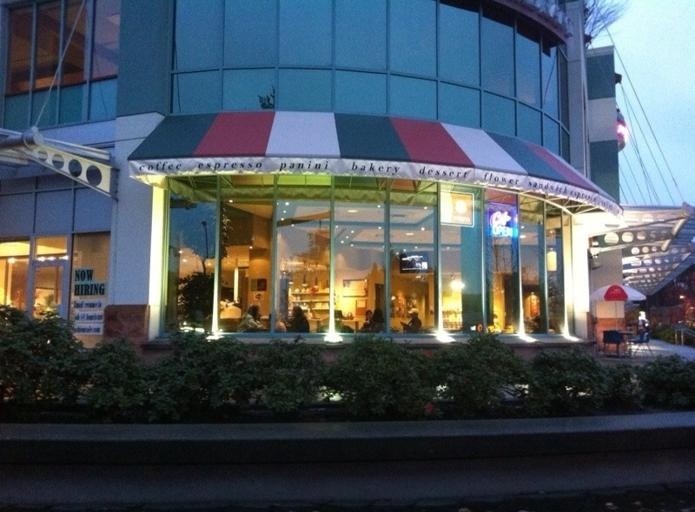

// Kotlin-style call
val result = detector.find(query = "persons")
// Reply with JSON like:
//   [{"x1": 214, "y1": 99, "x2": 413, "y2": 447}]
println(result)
[
  {"x1": 283, "y1": 306, "x2": 311, "y2": 333},
  {"x1": 362, "y1": 308, "x2": 386, "y2": 333},
  {"x1": 398, "y1": 309, "x2": 423, "y2": 332},
  {"x1": 363, "y1": 308, "x2": 371, "y2": 327},
  {"x1": 236, "y1": 304, "x2": 264, "y2": 334}
]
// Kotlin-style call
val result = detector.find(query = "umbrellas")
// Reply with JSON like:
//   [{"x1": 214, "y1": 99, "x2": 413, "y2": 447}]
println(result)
[{"x1": 592, "y1": 281, "x2": 649, "y2": 332}]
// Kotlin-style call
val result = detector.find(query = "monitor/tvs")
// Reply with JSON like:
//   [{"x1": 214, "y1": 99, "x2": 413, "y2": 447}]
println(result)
[{"x1": 400, "y1": 250, "x2": 432, "y2": 273}]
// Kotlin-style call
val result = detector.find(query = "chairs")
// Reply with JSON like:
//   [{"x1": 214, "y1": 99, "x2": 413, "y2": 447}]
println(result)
[{"x1": 603, "y1": 329, "x2": 653, "y2": 359}]
